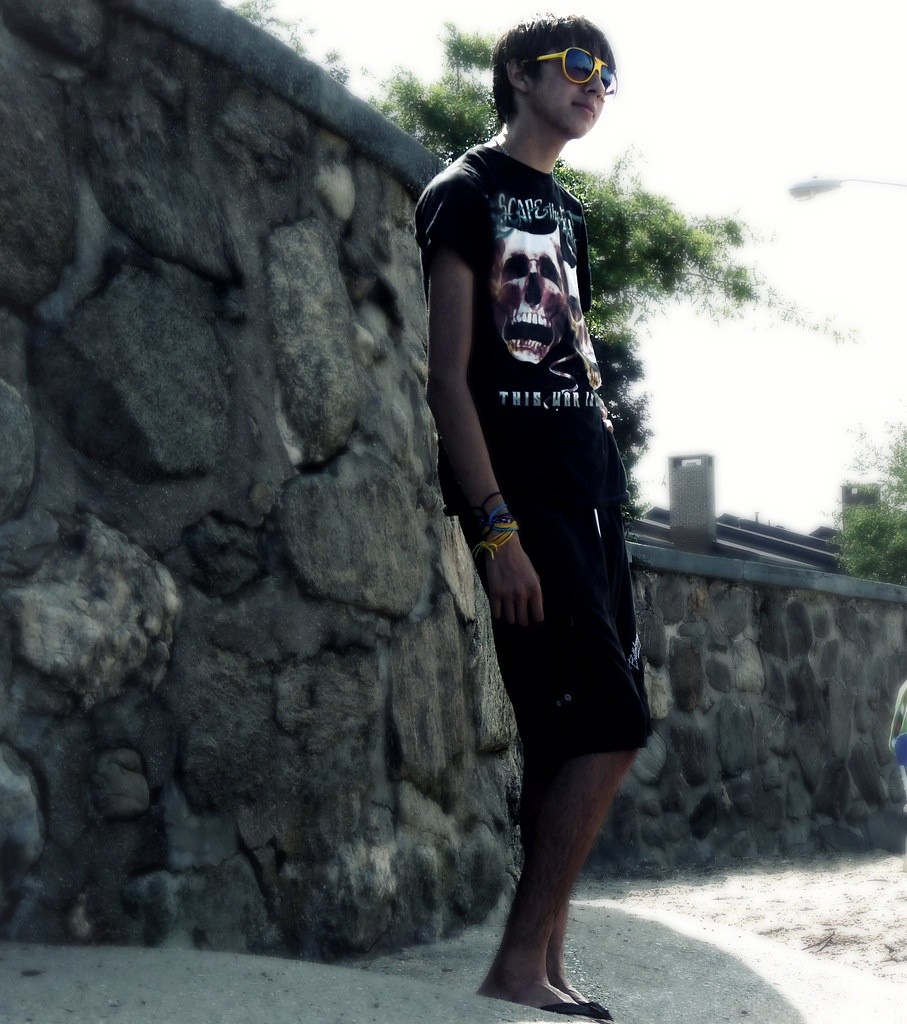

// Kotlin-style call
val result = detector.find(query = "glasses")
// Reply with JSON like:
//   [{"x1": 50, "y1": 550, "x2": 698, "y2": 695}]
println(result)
[{"x1": 522, "y1": 47, "x2": 618, "y2": 104}]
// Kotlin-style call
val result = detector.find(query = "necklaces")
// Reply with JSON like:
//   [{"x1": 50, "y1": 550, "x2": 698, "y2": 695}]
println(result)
[{"x1": 493, "y1": 135, "x2": 509, "y2": 157}]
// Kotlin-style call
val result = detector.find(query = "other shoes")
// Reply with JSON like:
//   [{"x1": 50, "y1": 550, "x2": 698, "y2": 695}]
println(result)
[{"x1": 539, "y1": 1001, "x2": 615, "y2": 1024}]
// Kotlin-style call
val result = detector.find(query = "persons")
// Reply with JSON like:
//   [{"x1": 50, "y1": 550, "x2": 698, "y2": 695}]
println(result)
[{"x1": 410, "y1": 15, "x2": 656, "y2": 1020}]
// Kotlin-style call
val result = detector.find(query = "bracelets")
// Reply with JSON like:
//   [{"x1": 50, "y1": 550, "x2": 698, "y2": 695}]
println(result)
[{"x1": 457, "y1": 492, "x2": 518, "y2": 561}]
